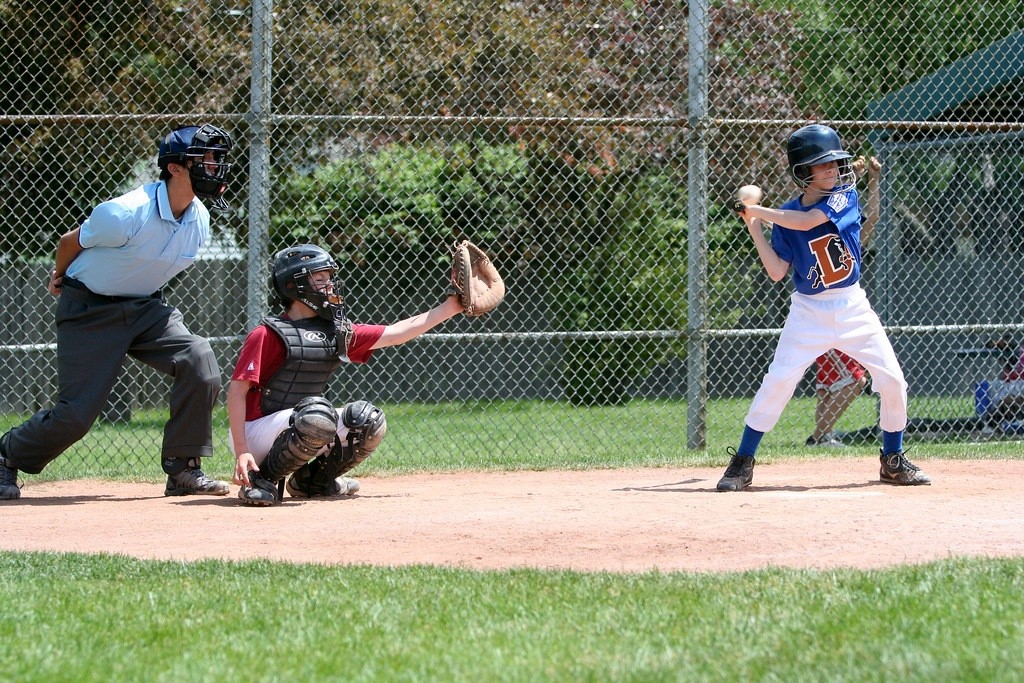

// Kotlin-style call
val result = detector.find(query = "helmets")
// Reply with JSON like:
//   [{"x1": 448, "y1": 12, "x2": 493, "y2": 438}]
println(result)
[
  {"x1": 786, "y1": 124, "x2": 852, "y2": 188},
  {"x1": 272, "y1": 243, "x2": 347, "y2": 324},
  {"x1": 157, "y1": 125, "x2": 228, "y2": 167}
]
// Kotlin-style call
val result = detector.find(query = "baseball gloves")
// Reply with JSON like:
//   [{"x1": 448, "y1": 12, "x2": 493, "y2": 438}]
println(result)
[{"x1": 447, "y1": 239, "x2": 506, "y2": 318}]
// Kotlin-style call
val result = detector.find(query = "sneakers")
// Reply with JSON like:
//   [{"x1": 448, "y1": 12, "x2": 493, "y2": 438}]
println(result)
[
  {"x1": 0, "y1": 432, "x2": 20, "y2": 499},
  {"x1": 164, "y1": 459, "x2": 230, "y2": 497},
  {"x1": 716, "y1": 446, "x2": 755, "y2": 491},
  {"x1": 879, "y1": 445, "x2": 931, "y2": 485},
  {"x1": 286, "y1": 465, "x2": 360, "y2": 496},
  {"x1": 237, "y1": 470, "x2": 273, "y2": 506}
]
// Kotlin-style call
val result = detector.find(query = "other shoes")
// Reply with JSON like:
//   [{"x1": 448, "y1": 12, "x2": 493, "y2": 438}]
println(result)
[{"x1": 806, "y1": 434, "x2": 844, "y2": 447}]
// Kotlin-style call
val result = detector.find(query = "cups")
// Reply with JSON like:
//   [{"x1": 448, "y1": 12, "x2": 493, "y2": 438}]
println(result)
[{"x1": 975, "y1": 382, "x2": 990, "y2": 416}]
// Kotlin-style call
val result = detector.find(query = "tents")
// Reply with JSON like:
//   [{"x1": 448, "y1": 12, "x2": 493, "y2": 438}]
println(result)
[{"x1": 868, "y1": 30, "x2": 1023, "y2": 439}]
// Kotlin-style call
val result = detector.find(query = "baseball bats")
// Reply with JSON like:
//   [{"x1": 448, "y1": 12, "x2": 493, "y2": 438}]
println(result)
[{"x1": 731, "y1": 199, "x2": 774, "y2": 230}]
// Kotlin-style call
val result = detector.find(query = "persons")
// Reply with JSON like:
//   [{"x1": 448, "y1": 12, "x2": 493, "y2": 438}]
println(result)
[
  {"x1": 717, "y1": 124, "x2": 931, "y2": 490},
  {"x1": 227, "y1": 241, "x2": 505, "y2": 506},
  {"x1": 0, "y1": 123, "x2": 233, "y2": 501}
]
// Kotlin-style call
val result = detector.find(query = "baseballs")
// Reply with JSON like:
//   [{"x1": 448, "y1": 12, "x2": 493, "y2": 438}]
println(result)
[{"x1": 737, "y1": 185, "x2": 762, "y2": 205}]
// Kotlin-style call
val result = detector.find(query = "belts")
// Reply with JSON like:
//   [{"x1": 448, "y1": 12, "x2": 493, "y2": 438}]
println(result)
[{"x1": 62, "y1": 277, "x2": 95, "y2": 294}]
